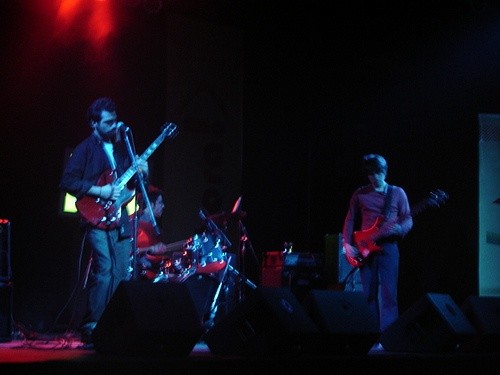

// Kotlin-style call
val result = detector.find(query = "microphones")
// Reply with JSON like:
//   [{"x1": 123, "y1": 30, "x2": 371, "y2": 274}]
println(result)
[{"x1": 117, "y1": 121, "x2": 129, "y2": 132}]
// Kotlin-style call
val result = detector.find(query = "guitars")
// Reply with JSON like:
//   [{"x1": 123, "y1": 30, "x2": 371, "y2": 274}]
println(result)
[
  {"x1": 75, "y1": 123, "x2": 178, "y2": 231},
  {"x1": 344, "y1": 189, "x2": 449, "y2": 267}
]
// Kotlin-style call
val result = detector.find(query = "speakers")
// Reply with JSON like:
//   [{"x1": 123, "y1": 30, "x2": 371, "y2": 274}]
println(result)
[
  {"x1": 90, "y1": 278, "x2": 206, "y2": 360},
  {"x1": 296, "y1": 290, "x2": 383, "y2": 357},
  {"x1": 200, "y1": 286, "x2": 318, "y2": 357},
  {"x1": 377, "y1": 292, "x2": 477, "y2": 357},
  {"x1": 461, "y1": 295, "x2": 500, "y2": 352},
  {"x1": 0, "y1": 219, "x2": 14, "y2": 343}
]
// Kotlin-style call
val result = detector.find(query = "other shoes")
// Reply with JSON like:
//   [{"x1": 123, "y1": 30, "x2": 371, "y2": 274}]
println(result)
[
  {"x1": 371, "y1": 343, "x2": 384, "y2": 352},
  {"x1": 82, "y1": 321, "x2": 98, "y2": 330}
]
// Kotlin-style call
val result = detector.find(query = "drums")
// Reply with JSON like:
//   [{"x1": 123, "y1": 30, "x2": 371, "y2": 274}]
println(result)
[
  {"x1": 189, "y1": 232, "x2": 227, "y2": 274},
  {"x1": 152, "y1": 270, "x2": 226, "y2": 326}
]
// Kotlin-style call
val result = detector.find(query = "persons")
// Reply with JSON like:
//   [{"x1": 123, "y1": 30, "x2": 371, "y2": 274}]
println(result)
[
  {"x1": 61, "y1": 98, "x2": 149, "y2": 343},
  {"x1": 342, "y1": 154, "x2": 413, "y2": 351},
  {"x1": 136, "y1": 186, "x2": 165, "y2": 279}
]
